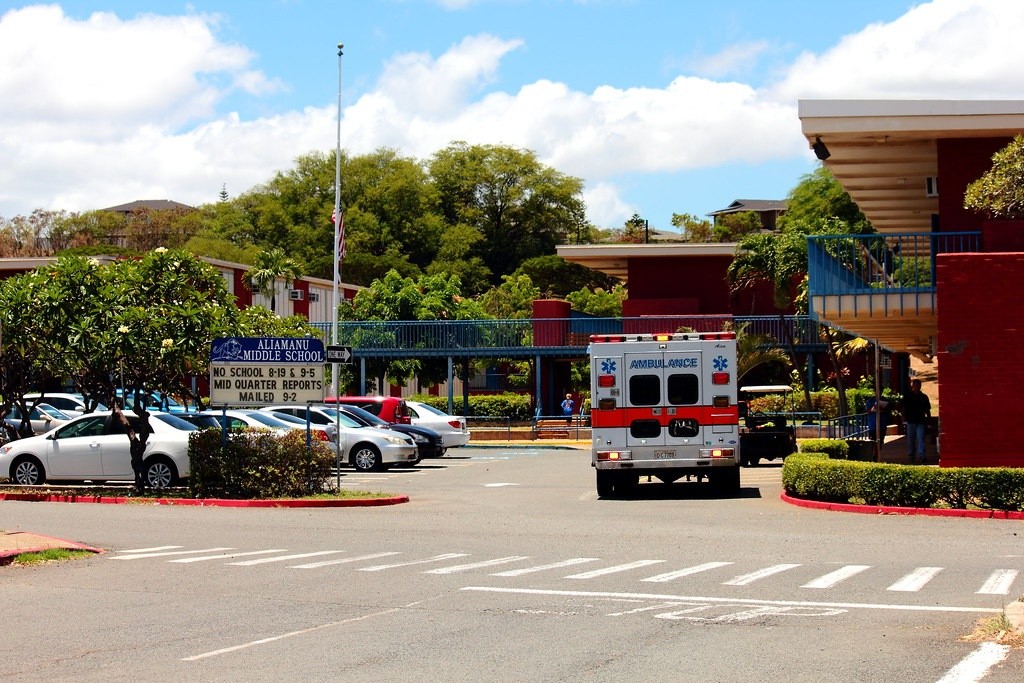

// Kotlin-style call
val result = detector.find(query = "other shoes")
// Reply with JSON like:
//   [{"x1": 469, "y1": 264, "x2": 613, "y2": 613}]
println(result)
[
  {"x1": 908, "y1": 455, "x2": 915, "y2": 464},
  {"x1": 919, "y1": 456, "x2": 928, "y2": 465}
]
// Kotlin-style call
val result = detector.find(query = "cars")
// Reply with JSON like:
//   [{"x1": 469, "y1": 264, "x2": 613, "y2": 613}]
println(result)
[
  {"x1": 0, "y1": 400, "x2": 75, "y2": 435},
  {"x1": 257, "y1": 409, "x2": 344, "y2": 464},
  {"x1": 323, "y1": 396, "x2": 411, "y2": 426},
  {"x1": 108, "y1": 387, "x2": 209, "y2": 424},
  {"x1": 176, "y1": 411, "x2": 316, "y2": 477},
  {"x1": 405, "y1": 402, "x2": 471, "y2": 456},
  {"x1": 0, "y1": 409, "x2": 208, "y2": 490},
  {"x1": 311, "y1": 403, "x2": 446, "y2": 470},
  {"x1": 258, "y1": 404, "x2": 420, "y2": 472},
  {"x1": 20, "y1": 393, "x2": 111, "y2": 424}
]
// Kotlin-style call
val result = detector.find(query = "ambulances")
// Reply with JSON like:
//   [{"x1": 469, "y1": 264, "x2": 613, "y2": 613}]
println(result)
[{"x1": 585, "y1": 331, "x2": 743, "y2": 496}]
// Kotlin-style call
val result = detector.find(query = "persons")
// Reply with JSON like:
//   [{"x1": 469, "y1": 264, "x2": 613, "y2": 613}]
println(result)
[
  {"x1": 866, "y1": 385, "x2": 889, "y2": 462},
  {"x1": 560, "y1": 393, "x2": 575, "y2": 427},
  {"x1": 900, "y1": 379, "x2": 932, "y2": 463},
  {"x1": 436, "y1": 310, "x2": 451, "y2": 347},
  {"x1": 31, "y1": 407, "x2": 40, "y2": 420}
]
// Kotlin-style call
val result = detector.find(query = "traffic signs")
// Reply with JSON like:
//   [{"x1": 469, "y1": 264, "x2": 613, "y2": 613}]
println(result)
[{"x1": 325, "y1": 344, "x2": 354, "y2": 364}]
